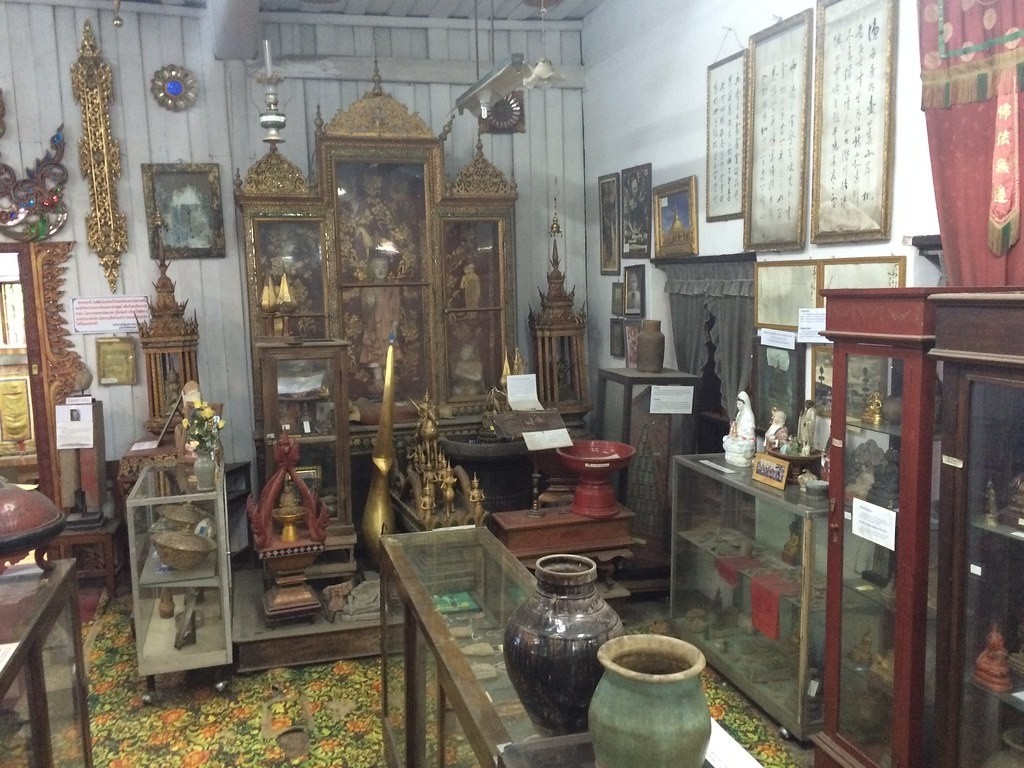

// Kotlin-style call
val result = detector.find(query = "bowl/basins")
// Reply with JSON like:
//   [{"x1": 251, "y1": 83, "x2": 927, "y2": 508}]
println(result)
[
  {"x1": 157, "y1": 505, "x2": 212, "y2": 529},
  {"x1": 155, "y1": 523, "x2": 216, "y2": 569}
]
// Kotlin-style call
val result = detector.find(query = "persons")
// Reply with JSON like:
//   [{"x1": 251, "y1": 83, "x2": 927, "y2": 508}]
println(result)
[
  {"x1": 407, "y1": 388, "x2": 485, "y2": 531},
  {"x1": 849, "y1": 390, "x2": 1024, "y2": 690},
  {"x1": 722, "y1": 390, "x2": 756, "y2": 453},
  {"x1": 762, "y1": 399, "x2": 817, "y2": 457},
  {"x1": 359, "y1": 252, "x2": 406, "y2": 391},
  {"x1": 458, "y1": 263, "x2": 481, "y2": 320},
  {"x1": 450, "y1": 343, "x2": 483, "y2": 396}
]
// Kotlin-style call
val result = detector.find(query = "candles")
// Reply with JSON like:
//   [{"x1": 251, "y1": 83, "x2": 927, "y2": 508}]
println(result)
[{"x1": 263, "y1": 38, "x2": 274, "y2": 78}]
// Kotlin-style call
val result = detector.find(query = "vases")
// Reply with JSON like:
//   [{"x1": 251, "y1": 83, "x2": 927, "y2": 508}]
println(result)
[{"x1": 193, "y1": 456, "x2": 216, "y2": 491}]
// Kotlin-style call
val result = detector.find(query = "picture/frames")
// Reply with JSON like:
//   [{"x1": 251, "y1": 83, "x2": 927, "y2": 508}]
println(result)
[
  {"x1": 597, "y1": 173, "x2": 621, "y2": 276},
  {"x1": 609, "y1": 317, "x2": 624, "y2": 358},
  {"x1": 653, "y1": 174, "x2": 699, "y2": 258},
  {"x1": 0, "y1": 277, "x2": 26, "y2": 346},
  {"x1": 743, "y1": 9, "x2": 811, "y2": 254},
  {"x1": 704, "y1": 48, "x2": 746, "y2": 221},
  {"x1": 809, "y1": 343, "x2": 889, "y2": 423},
  {"x1": 623, "y1": 265, "x2": 646, "y2": 318},
  {"x1": 812, "y1": 0, "x2": 900, "y2": 246},
  {"x1": 751, "y1": 335, "x2": 806, "y2": 438},
  {"x1": 621, "y1": 162, "x2": 651, "y2": 259},
  {"x1": 751, "y1": 452, "x2": 789, "y2": 490},
  {"x1": 611, "y1": 280, "x2": 624, "y2": 316},
  {"x1": 624, "y1": 319, "x2": 642, "y2": 368},
  {"x1": 140, "y1": 161, "x2": 227, "y2": 259},
  {"x1": 94, "y1": 336, "x2": 136, "y2": 384}
]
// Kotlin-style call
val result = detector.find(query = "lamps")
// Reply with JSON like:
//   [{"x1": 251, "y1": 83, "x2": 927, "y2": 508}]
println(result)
[{"x1": 523, "y1": 0, "x2": 566, "y2": 93}]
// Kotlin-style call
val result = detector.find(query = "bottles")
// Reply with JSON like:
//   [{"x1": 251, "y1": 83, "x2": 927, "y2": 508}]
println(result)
[
  {"x1": 588, "y1": 634, "x2": 712, "y2": 768},
  {"x1": 637, "y1": 319, "x2": 665, "y2": 372},
  {"x1": 504, "y1": 553, "x2": 624, "y2": 729}
]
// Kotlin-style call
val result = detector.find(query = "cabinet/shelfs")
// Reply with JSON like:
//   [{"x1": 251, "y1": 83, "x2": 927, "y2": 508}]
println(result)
[
  {"x1": 930, "y1": 292, "x2": 1024, "y2": 768},
  {"x1": 377, "y1": 526, "x2": 762, "y2": 768},
  {"x1": 123, "y1": 459, "x2": 232, "y2": 677},
  {"x1": 667, "y1": 453, "x2": 807, "y2": 742},
  {"x1": 1, "y1": 557, "x2": 93, "y2": 768},
  {"x1": 256, "y1": 340, "x2": 354, "y2": 540},
  {"x1": 812, "y1": 286, "x2": 925, "y2": 768}
]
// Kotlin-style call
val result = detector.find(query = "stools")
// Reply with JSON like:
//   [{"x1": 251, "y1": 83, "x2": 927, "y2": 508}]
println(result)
[{"x1": 53, "y1": 522, "x2": 123, "y2": 601}]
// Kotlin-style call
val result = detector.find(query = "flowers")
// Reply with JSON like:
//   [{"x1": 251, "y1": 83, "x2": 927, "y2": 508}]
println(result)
[{"x1": 181, "y1": 399, "x2": 225, "y2": 454}]
